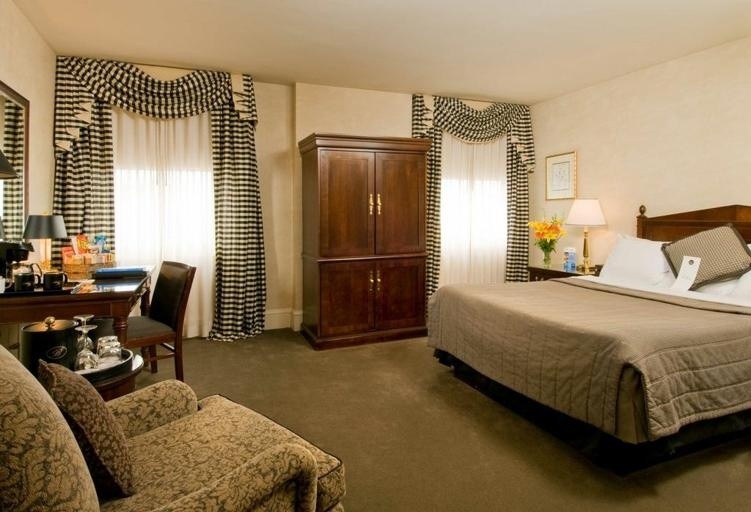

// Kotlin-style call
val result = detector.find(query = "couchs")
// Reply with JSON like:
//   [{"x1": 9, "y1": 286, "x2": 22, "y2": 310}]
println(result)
[{"x1": 1, "y1": 340, "x2": 345, "y2": 511}]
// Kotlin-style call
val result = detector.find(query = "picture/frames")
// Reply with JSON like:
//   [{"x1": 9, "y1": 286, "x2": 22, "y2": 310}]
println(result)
[{"x1": 546, "y1": 152, "x2": 581, "y2": 201}]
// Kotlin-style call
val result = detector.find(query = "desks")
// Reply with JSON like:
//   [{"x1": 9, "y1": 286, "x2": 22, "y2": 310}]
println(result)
[
  {"x1": 89, "y1": 351, "x2": 146, "y2": 402},
  {"x1": 1, "y1": 260, "x2": 155, "y2": 368}
]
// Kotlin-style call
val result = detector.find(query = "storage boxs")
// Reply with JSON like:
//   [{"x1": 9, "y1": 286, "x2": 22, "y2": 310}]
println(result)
[{"x1": 61, "y1": 263, "x2": 114, "y2": 281}]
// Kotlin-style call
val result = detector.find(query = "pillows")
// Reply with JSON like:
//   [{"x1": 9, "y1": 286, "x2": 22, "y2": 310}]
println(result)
[
  {"x1": 695, "y1": 245, "x2": 750, "y2": 298},
  {"x1": 603, "y1": 235, "x2": 671, "y2": 288},
  {"x1": 34, "y1": 355, "x2": 135, "y2": 498},
  {"x1": 658, "y1": 222, "x2": 751, "y2": 292}
]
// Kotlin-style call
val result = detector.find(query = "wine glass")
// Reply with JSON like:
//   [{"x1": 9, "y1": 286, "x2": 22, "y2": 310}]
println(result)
[
  {"x1": 74, "y1": 314, "x2": 94, "y2": 351},
  {"x1": 73, "y1": 324, "x2": 97, "y2": 370}
]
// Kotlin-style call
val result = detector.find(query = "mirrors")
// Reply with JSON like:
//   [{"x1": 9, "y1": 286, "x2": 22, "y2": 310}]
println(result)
[{"x1": 0, "y1": 82, "x2": 28, "y2": 248}]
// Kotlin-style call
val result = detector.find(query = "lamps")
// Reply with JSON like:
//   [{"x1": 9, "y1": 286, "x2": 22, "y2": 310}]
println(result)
[
  {"x1": 0, "y1": 149, "x2": 18, "y2": 182},
  {"x1": 21, "y1": 213, "x2": 68, "y2": 270},
  {"x1": 565, "y1": 197, "x2": 607, "y2": 274}
]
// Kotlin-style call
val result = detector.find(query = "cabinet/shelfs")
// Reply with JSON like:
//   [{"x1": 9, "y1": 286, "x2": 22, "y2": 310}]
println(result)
[{"x1": 298, "y1": 133, "x2": 430, "y2": 350}]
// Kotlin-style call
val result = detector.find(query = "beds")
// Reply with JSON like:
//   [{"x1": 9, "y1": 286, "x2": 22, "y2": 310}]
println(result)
[{"x1": 430, "y1": 198, "x2": 751, "y2": 474}]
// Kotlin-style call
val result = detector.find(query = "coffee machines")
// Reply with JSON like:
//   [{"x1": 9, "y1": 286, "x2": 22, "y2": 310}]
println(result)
[{"x1": 0, "y1": 241, "x2": 43, "y2": 292}]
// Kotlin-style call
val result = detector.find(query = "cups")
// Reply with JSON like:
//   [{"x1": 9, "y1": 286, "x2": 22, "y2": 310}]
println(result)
[
  {"x1": 17, "y1": 272, "x2": 40, "y2": 290},
  {"x1": 43, "y1": 271, "x2": 69, "y2": 291},
  {"x1": 0, "y1": 277, "x2": 13, "y2": 294},
  {"x1": 99, "y1": 335, "x2": 121, "y2": 366}
]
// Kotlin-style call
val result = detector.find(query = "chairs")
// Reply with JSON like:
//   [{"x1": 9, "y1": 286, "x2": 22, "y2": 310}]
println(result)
[{"x1": 116, "y1": 263, "x2": 196, "y2": 384}]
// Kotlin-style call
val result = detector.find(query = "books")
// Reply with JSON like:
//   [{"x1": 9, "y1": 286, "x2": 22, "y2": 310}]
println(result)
[{"x1": 92, "y1": 266, "x2": 147, "y2": 283}]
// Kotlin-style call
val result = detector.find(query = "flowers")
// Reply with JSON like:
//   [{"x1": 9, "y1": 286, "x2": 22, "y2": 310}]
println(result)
[{"x1": 525, "y1": 214, "x2": 564, "y2": 266}]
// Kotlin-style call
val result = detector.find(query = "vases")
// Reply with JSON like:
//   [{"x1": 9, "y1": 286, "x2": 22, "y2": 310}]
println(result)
[{"x1": 543, "y1": 251, "x2": 550, "y2": 270}]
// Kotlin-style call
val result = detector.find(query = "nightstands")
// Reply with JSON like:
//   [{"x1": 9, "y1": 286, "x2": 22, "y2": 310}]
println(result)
[{"x1": 523, "y1": 266, "x2": 595, "y2": 281}]
